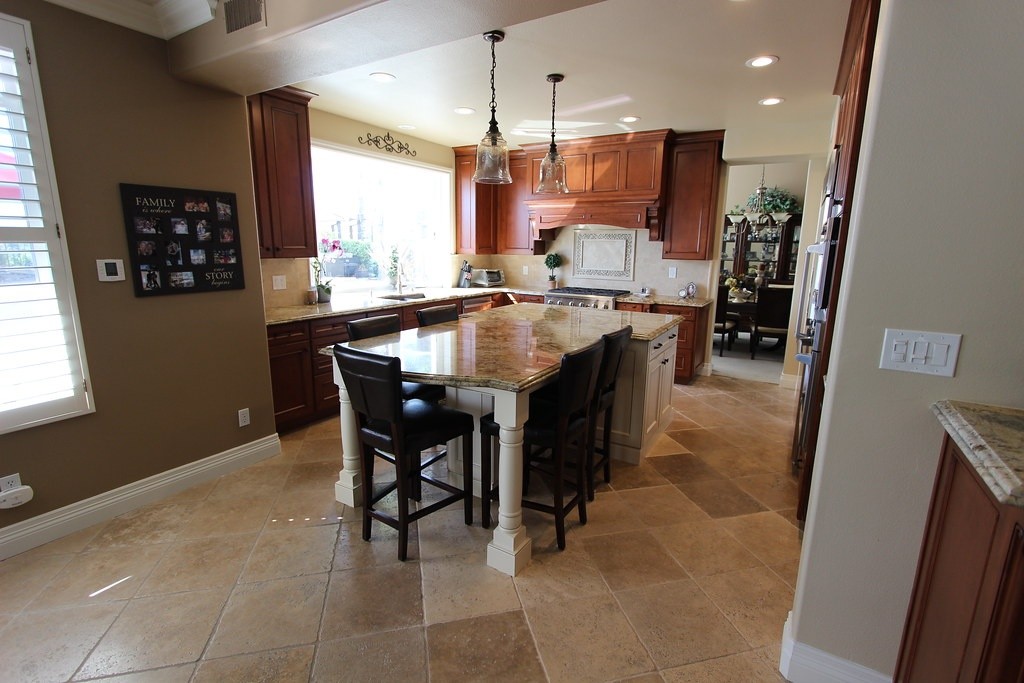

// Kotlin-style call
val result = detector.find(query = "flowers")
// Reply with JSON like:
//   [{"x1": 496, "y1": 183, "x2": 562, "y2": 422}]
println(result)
[{"x1": 311, "y1": 238, "x2": 344, "y2": 293}]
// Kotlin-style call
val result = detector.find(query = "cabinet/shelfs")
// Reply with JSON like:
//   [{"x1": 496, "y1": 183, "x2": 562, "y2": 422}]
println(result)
[
  {"x1": 452, "y1": 128, "x2": 730, "y2": 260},
  {"x1": 266, "y1": 295, "x2": 543, "y2": 578},
  {"x1": 720, "y1": 213, "x2": 802, "y2": 332},
  {"x1": 246, "y1": 85, "x2": 319, "y2": 259},
  {"x1": 785, "y1": 0, "x2": 882, "y2": 519},
  {"x1": 617, "y1": 302, "x2": 710, "y2": 385}
]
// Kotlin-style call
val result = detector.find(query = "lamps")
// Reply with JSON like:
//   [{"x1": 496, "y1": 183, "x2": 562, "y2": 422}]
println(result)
[
  {"x1": 535, "y1": 74, "x2": 570, "y2": 194},
  {"x1": 472, "y1": 30, "x2": 513, "y2": 185},
  {"x1": 727, "y1": 163, "x2": 803, "y2": 234}
]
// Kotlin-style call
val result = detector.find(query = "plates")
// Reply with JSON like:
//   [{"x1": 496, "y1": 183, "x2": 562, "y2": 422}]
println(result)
[
  {"x1": 678, "y1": 288, "x2": 687, "y2": 297},
  {"x1": 685, "y1": 282, "x2": 697, "y2": 296}
]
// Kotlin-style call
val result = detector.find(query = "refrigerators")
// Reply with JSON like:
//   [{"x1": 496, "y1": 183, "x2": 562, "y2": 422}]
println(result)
[{"x1": 791, "y1": 148, "x2": 842, "y2": 474}]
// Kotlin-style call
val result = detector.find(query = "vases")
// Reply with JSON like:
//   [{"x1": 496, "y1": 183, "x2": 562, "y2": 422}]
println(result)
[{"x1": 317, "y1": 285, "x2": 331, "y2": 303}]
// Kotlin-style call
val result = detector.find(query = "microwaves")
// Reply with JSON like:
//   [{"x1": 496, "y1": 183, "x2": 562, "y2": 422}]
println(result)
[{"x1": 470, "y1": 269, "x2": 506, "y2": 287}]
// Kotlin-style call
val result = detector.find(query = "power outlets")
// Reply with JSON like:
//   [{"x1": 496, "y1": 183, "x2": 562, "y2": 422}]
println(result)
[
  {"x1": 238, "y1": 408, "x2": 250, "y2": 428},
  {"x1": 272, "y1": 275, "x2": 287, "y2": 290},
  {"x1": 0, "y1": 473, "x2": 22, "y2": 491}
]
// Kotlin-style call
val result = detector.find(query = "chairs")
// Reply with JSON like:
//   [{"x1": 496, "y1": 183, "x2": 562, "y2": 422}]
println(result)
[
  {"x1": 717, "y1": 275, "x2": 795, "y2": 362},
  {"x1": 333, "y1": 304, "x2": 633, "y2": 562}
]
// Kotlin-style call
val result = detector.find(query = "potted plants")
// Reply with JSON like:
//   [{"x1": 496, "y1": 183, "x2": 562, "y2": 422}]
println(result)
[
  {"x1": 387, "y1": 244, "x2": 399, "y2": 290},
  {"x1": 544, "y1": 253, "x2": 562, "y2": 289}
]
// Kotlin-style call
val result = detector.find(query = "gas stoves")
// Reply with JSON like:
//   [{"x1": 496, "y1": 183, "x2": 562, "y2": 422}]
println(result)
[{"x1": 545, "y1": 286, "x2": 632, "y2": 310}]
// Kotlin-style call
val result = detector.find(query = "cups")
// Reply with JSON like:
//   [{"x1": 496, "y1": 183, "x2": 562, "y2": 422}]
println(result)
[{"x1": 308, "y1": 290, "x2": 316, "y2": 305}]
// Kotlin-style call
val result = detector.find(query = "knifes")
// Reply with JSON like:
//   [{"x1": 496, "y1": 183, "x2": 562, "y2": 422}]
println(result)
[{"x1": 461, "y1": 260, "x2": 471, "y2": 273}]
